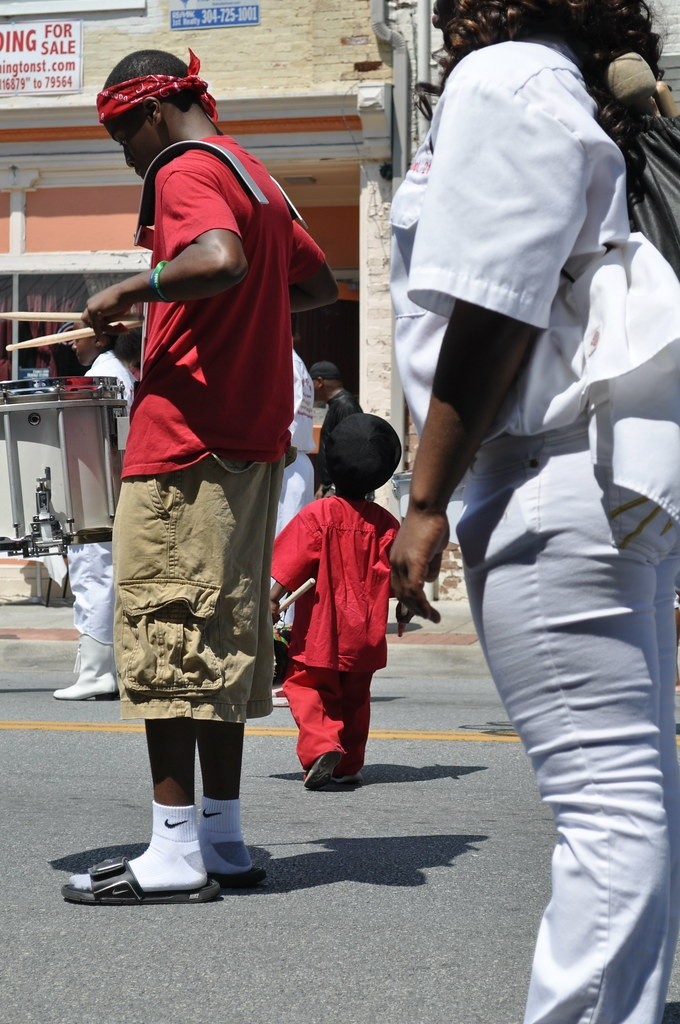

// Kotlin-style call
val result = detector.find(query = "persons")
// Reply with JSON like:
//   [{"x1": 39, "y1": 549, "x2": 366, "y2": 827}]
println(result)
[
  {"x1": 53, "y1": 321, "x2": 134, "y2": 701},
  {"x1": 310, "y1": 362, "x2": 363, "y2": 500},
  {"x1": 61, "y1": 48, "x2": 341, "y2": 906},
  {"x1": 270, "y1": 349, "x2": 315, "y2": 707},
  {"x1": 389, "y1": 0, "x2": 680, "y2": 1024},
  {"x1": 270, "y1": 414, "x2": 416, "y2": 789}
]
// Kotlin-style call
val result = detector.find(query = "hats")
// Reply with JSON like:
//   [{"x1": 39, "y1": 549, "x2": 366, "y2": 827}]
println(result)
[
  {"x1": 308, "y1": 361, "x2": 340, "y2": 380},
  {"x1": 325, "y1": 414, "x2": 402, "y2": 493}
]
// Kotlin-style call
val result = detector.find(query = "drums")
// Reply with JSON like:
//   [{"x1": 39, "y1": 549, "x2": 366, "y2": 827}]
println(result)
[{"x1": 0, "y1": 374, "x2": 129, "y2": 553}]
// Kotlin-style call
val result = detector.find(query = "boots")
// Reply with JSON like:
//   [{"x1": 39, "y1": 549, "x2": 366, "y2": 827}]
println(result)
[{"x1": 53, "y1": 634, "x2": 120, "y2": 700}]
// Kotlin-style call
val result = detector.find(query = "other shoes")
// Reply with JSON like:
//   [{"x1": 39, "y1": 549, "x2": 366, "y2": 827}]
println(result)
[
  {"x1": 330, "y1": 767, "x2": 362, "y2": 783},
  {"x1": 270, "y1": 690, "x2": 292, "y2": 707},
  {"x1": 303, "y1": 751, "x2": 342, "y2": 789}
]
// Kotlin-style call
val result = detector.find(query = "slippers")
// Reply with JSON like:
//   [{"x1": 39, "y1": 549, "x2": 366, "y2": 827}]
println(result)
[
  {"x1": 205, "y1": 867, "x2": 267, "y2": 886},
  {"x1": 61, "y1": 856, "x2": 221, "y2": 905}
]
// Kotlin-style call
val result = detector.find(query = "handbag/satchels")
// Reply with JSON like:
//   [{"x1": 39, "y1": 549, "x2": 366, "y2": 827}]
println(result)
[{"x1": 616, "y1": 112, "x2": 680, "y2": 282}]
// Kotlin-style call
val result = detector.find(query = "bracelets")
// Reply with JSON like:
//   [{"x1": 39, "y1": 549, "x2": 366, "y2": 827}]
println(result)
[{"x1": 150, "y1": 260, "x2": 174, "y2": 302}]
